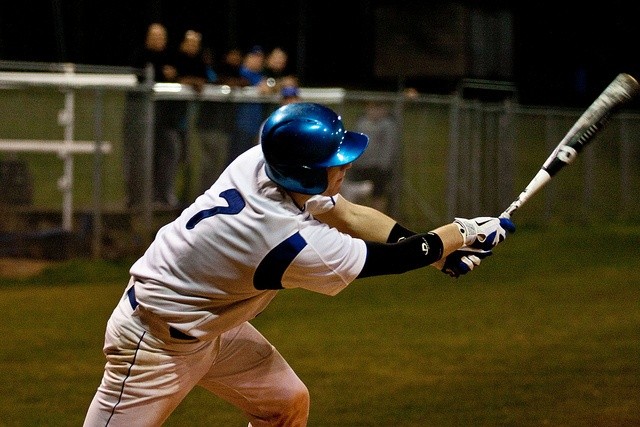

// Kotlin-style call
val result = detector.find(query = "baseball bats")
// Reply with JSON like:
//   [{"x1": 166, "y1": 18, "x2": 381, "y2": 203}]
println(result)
[{"x1": 498, "y1": 72, "x2": 639, "y2": 219}]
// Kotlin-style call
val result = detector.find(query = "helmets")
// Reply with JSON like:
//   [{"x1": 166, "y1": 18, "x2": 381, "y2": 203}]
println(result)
[{"x1": 261, "y1": 103, "x2": 369, "y2": 195}]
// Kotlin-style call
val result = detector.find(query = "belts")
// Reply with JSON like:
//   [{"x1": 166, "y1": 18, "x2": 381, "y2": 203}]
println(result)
[{"x1": 128, "y1": 286, "x2": 197, "y2": 340}]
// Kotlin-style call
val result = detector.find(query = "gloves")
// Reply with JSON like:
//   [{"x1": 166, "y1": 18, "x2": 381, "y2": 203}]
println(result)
[
  {"x1": 431, "y1": 248, "x2": 492, "y2": 279},
  {"x1": 452, "y1": 217, "x2": 515, "y2": 251}
]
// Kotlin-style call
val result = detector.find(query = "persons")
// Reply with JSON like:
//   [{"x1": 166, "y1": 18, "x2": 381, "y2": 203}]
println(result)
[
  {"x1": 80, "y1": 101, "x2": 519, "y2": 427},
  {"x1": 349, "y1": 101, "x2": 401, "y2": 213},
  {"x1": 131, "y1": 20, "x2": 302, "y2": 206}
]
